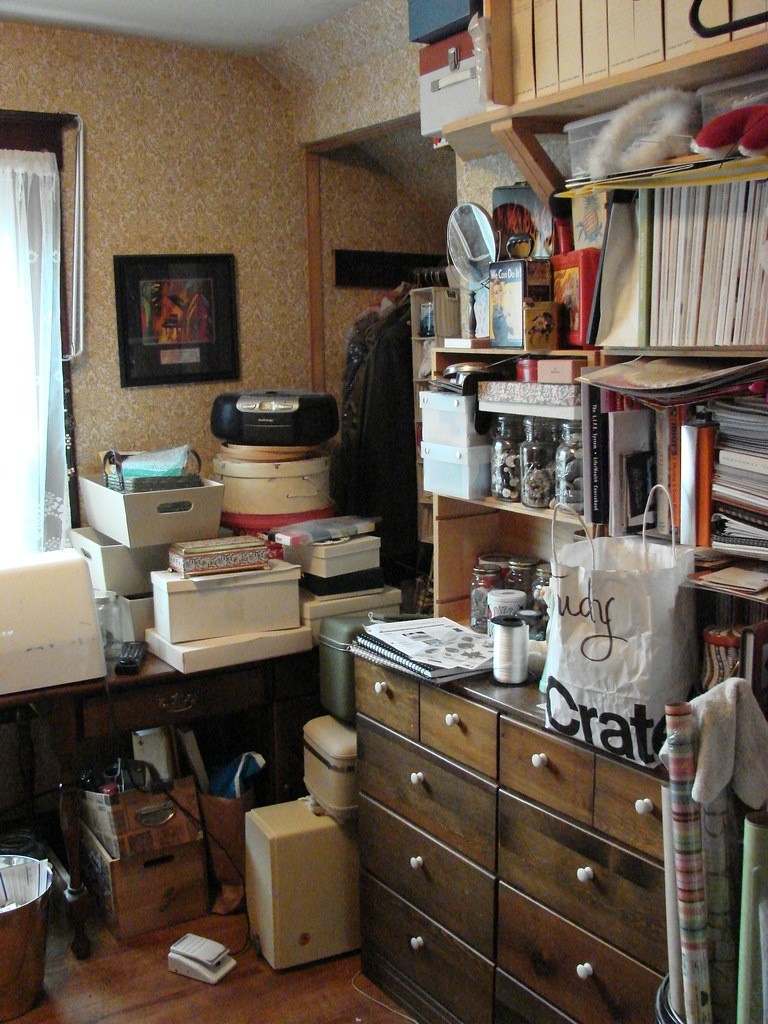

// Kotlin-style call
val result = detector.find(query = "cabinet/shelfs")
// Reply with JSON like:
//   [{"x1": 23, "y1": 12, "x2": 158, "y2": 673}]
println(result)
[{"x1": 347, "y1": 0, "x2": 768, "y2": 1024}]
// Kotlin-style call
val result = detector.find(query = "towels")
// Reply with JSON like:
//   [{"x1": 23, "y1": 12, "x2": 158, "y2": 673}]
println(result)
[{"x1": 655, "y1": 676, "x2": 768, "y2": 810}]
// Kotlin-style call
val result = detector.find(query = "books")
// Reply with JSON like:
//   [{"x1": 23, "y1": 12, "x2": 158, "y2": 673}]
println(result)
[
  {"x1": 573, "y1": 348, "x2": 768, "y2": 600},
  {"x1": 595, "y1": 180, "x2": 768, "y2": 349},
  {"x1": 345, "y1": 629, "x2": 493, "y2": 684},
  {"x1": 712, "y1": 591, "x2": 768, "y2": 713}
]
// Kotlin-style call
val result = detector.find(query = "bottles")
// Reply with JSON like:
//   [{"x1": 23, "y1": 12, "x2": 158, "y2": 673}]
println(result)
[
  {"x1": 515, "y1": 609, "x2": 546, "y2": 641},
  {"x1": 519, "y1": 417, "x2": 561, "y2": 508},
  {"x1": 94, "y1": 591, "x2": 122, "y2": 659},
  {"x1": 469, "y1": 564, "x2": 504, "y2": 633},
  {"x1": 490, "y1": 417, "x2": 526, "y2": 503},
  {"x1": 555, "y1": 421, "x2": 584, "y2": 516},
  {"x1": 531, "y1": 564, "x2": 552, "y2": 624},
  {"x1": 504, "y1": 556, "x2": 541, "y2": 609}
]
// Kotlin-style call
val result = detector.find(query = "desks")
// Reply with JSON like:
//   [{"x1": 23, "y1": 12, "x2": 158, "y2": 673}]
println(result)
[{"x1": 0, "y1": 655, "x2": 327, "y2": 958}]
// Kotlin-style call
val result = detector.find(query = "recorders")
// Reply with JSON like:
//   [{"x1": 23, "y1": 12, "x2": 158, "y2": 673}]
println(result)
[{"x1": 209, "y1": 388, "x2": 339, "y2": 447}]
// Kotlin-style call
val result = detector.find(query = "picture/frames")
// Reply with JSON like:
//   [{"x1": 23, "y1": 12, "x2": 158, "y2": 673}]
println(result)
[{"x1": 113, "y1": 254, "x2": 242, "y2": 388}]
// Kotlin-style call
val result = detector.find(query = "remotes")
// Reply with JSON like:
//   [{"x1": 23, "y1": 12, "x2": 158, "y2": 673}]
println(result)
[{"x1": 113, "y1": 641, "x2": 149, "y2": 676}]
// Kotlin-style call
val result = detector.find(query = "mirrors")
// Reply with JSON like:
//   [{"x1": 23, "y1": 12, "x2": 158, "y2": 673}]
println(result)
[{"x1": 445, "y1": 202, "x2": 502, "y2": 293}]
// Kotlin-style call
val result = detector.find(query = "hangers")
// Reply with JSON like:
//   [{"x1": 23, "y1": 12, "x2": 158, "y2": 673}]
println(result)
[{"x1": 351, "y1": 269, "x2": 449, "y2": 341}]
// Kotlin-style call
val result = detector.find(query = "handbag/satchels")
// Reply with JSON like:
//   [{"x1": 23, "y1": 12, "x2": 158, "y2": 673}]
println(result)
[{"x1": 547, "y1": 485, "x2": 698, "y2": 766}]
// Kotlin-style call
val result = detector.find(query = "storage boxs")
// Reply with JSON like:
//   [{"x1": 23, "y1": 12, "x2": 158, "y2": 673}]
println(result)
[
  {"x1": 561, "y1": 90, "x2": 698, "y2": 177},
  {"x1": 0, "y1": 476, "x2": 401, "y2": 969},
  {"x1": 695, "y1": 71, "x2": 768, "y2": 127},
  {"x1": 405, "y1": 0, "x2": 486, "y2": 142},
  {"x1": 420, "y1": 235, "x2": 599, "y2": 503}
]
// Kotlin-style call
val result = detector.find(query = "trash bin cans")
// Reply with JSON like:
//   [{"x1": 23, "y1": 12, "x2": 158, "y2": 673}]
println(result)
[{"x1": 0, "y1": 855, "x2": 53, "y2": 1022}]
